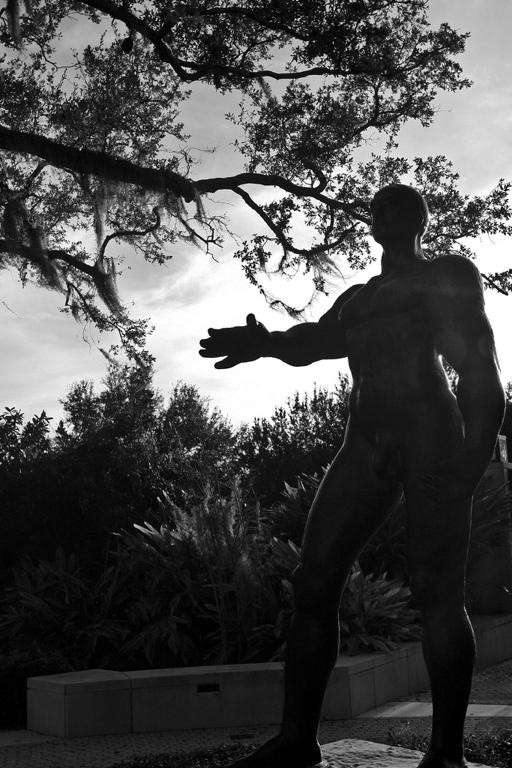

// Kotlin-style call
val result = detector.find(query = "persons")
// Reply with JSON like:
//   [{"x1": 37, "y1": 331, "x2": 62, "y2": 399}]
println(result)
[{"x1": 198, "y1": 182, "x2": 508, "y2": 768}]
